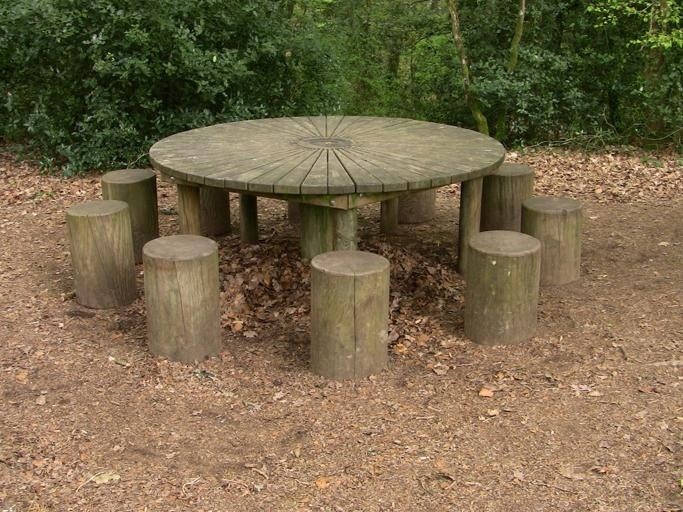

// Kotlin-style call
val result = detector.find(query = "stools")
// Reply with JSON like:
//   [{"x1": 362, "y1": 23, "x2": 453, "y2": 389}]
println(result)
[
  {"x1": 311, "y1": 251, "x2": 388, "y2": 379},
  {"x1": 288, "y1": 201, "x2": 307, "y2": 225},
  {"x1": 464, "y1": 231, "x2": 541, "y2": 346},
  {"x1": 177, "y1": 184, "x2": 231, "y2": 239},
  {"x1": 481, "y1": 163, "x2": 535, "y2": 232},
  {"x1": 67, "y1": 199, "x2": 136, "y2": 308},
  {"x1": 521, "y1": 197, "x2": 581, "y2": 285},
  {"x1": 101, "y1": 169, "x2": 158, "y2": 265},
  {"x1": 142, "y1": 235, "x2": 221, "y2": 362},
  {"x1": 400, "y1": 189, "x2": 436, "y2": 223}
]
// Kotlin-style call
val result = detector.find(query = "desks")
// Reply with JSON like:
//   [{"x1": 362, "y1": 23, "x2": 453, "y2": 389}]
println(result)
[{"x1": 150, "y1": 115, "x2": 506, "y2": 277}]
